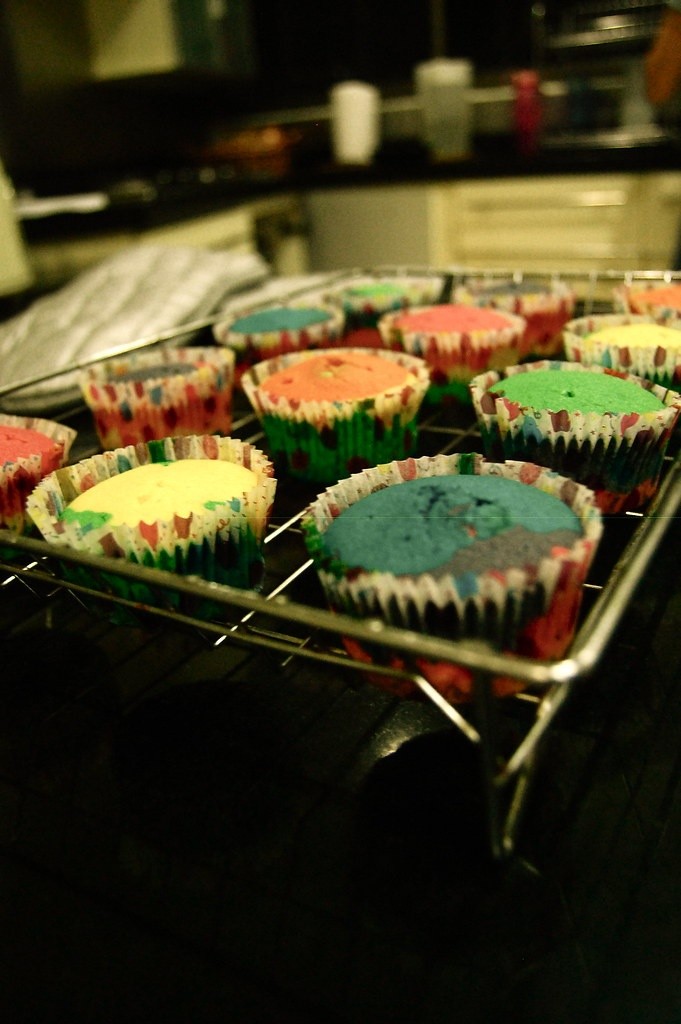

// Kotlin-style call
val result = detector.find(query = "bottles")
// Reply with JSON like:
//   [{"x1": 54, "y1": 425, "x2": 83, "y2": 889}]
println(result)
[
  {"x1": 1, "y1": 162, "x2": 36, "y2": 296},
  {"x1": 511, "y1": 70, "x2": 543, "y2": 135}
]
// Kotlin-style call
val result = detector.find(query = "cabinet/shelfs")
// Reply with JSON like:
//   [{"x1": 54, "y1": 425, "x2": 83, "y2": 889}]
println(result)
[{"x1": 29, "y1": 172, "x2": 680, "y2": 300}]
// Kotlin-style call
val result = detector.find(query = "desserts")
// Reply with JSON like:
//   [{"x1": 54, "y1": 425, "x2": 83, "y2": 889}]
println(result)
[{"x1": 0, "y1": 275, "x2": 681, "y2": 706}]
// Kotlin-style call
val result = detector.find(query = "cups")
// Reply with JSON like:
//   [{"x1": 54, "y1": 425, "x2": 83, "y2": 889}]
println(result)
[
  {"x1": 416, "y1": 61, "x2": 474, "y2": 159},
  {"x1": 329, "y1": 79, "x2": 383, "y2": 165}
]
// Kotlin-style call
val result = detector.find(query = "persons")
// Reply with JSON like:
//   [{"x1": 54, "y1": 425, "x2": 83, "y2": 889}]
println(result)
[{"x1": 641, "y1": 1, "x2": 681, "y2": 270}]
